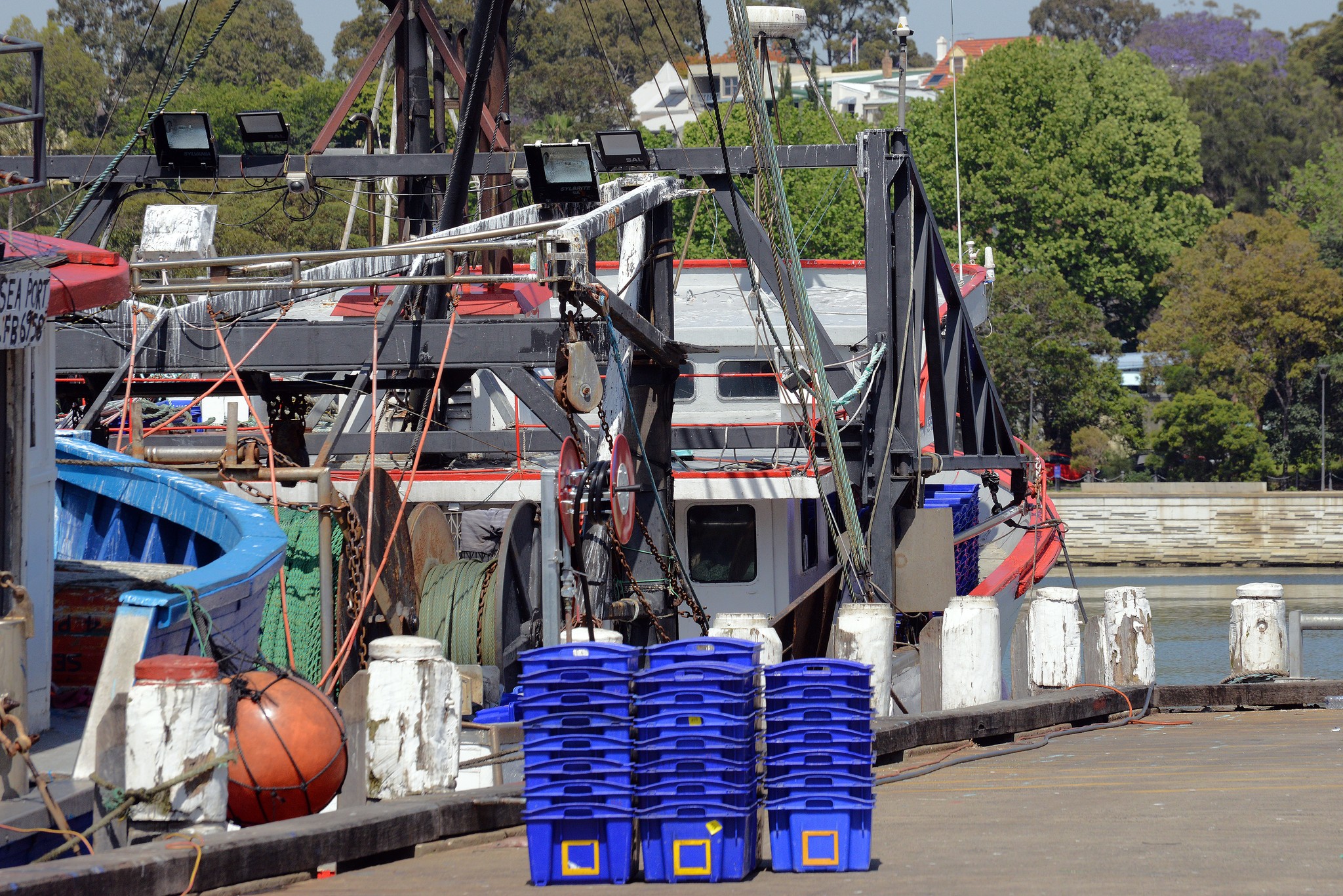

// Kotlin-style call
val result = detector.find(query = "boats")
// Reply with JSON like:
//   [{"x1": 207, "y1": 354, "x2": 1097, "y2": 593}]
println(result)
[{"x1": 0, "y1": 0, "x2": 1089, "y2": 896}]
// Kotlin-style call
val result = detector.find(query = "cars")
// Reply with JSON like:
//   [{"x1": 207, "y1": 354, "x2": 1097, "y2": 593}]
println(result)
[{"x1": 1026, "y1": 452, "x2": 1101, "y2": 487}]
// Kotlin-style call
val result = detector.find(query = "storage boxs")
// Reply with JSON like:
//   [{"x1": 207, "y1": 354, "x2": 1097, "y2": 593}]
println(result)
[
  {"x1": 473, "y1": 701, "x2": 521, "y2": 724},
  {"x1": 517, "y1": 637, "x2": 876, "y2": 886}
]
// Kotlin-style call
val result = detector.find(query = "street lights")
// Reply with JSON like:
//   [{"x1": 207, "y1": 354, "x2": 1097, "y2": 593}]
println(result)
[{"x1": 1316, "y1": 361, "x2": 1331, "y2": 491}]
